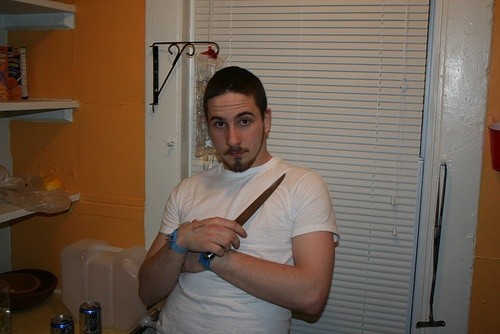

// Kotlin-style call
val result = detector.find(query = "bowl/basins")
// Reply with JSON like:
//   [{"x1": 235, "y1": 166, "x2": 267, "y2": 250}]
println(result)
[{"x1": 0, "y1": 269, "x2": 58, "y2": 310}]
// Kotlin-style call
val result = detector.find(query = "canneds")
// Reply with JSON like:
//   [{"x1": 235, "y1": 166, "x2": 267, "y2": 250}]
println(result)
[
  {"x1": 50, "y1": 314, "x2": 74, "y2": 334},
  {"x1": 79, "y1": 301, "x2": 102, "y2": 334}
]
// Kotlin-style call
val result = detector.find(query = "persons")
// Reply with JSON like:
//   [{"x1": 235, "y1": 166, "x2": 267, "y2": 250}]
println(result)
[{"x1": 138, "y1": 66, "x2": 340, "y2": 334}]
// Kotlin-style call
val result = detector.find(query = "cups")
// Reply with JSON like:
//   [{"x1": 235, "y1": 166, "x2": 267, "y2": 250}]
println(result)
[{"x1": 0, "y1": 279, "x2": 12, "y2": 334}]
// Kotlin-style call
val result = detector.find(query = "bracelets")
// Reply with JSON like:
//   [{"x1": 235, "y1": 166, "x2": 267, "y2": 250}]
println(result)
[
  {"x1": 166, "y1": 227, "x2": 190, "y2": 253},
  {"x1": 198, "y1": 251, "x2": 216, "y2": 271}
]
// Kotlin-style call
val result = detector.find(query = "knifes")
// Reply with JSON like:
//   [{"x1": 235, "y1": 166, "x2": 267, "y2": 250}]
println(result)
[{"x1": 204, "y1": 173, "x2": 285, "y2": 258}]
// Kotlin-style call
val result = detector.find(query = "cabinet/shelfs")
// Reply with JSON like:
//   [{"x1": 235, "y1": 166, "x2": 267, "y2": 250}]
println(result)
[{"x1": 0, "y1": 0, "x2": 81, "y2": 278}]
